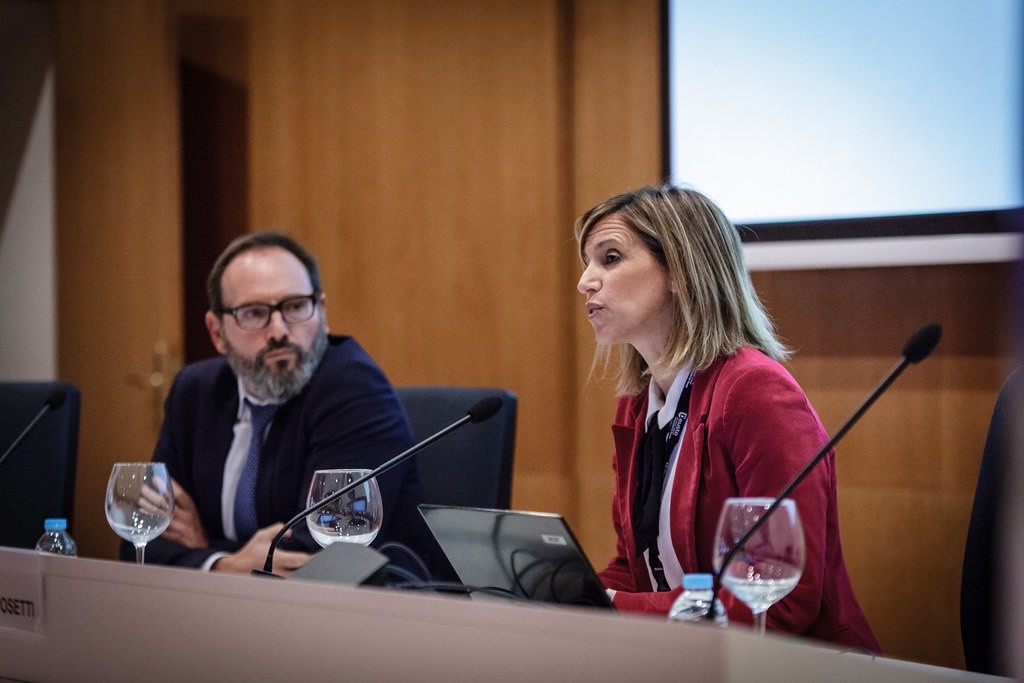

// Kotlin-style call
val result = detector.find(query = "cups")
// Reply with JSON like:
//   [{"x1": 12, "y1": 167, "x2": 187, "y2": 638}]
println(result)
[{"x1": 305, "y1": 468, "x2": 384, "y2": 550}]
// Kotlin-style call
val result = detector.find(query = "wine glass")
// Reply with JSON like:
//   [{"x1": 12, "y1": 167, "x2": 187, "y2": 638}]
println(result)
[
  {"x1": 103, "y1": 461, "x2": 176, "y2": 566},
  {"x1": 711, "y1": 496, "x2": 806, "y2": 638}
]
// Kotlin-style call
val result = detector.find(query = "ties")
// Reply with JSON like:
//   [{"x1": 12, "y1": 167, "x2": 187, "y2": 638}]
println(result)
[{"x1": 234, "y1": 398, "x2": 280, "y2": 545}]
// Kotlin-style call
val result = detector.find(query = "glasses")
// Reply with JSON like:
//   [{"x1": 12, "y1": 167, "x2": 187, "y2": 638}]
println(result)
[{"x1": 218, "y1": 287, "x2": 319, "y2": 331}]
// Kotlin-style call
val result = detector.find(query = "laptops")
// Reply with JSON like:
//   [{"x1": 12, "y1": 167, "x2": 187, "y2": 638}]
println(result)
[{"x1": 418, "y1": 503, "x2": 616, "y2": 610}]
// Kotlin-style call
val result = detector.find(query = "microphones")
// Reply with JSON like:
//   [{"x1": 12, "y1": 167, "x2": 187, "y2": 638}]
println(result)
[
  {"x1": 706, "y1": 323, "x2": 940, "y2": 617},
  {"x1": 252, "y1": 394, "x2": 503, "y2": 577},
  {"x1": 0, "y1": 386, "x2": 68, "y2": 465}
]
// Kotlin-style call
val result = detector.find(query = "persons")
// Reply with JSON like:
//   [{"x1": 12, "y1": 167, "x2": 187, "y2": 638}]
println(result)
[
  {"x1": 118, "y1": 231, "x2": 415, "y2": 578},
  {"x1": 574, "y1": 185, "x2": 882, "y2": 657}
]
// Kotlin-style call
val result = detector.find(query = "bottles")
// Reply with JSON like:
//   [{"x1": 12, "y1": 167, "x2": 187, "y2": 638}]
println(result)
[
  {"x1": 34, "y1": 517, "x2": 77, "y2": 557},
  {"x1": 666, "y1": 573, "x2": 729, "y2": 632}
]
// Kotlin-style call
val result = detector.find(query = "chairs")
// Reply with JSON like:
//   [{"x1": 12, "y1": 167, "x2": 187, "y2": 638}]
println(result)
[
  {"x1": 394, "y1": 386, "x2": 517, "y2": 594},
  {"x1": 0, "y1": 380, "x2": 81, "y2": 551}
]
